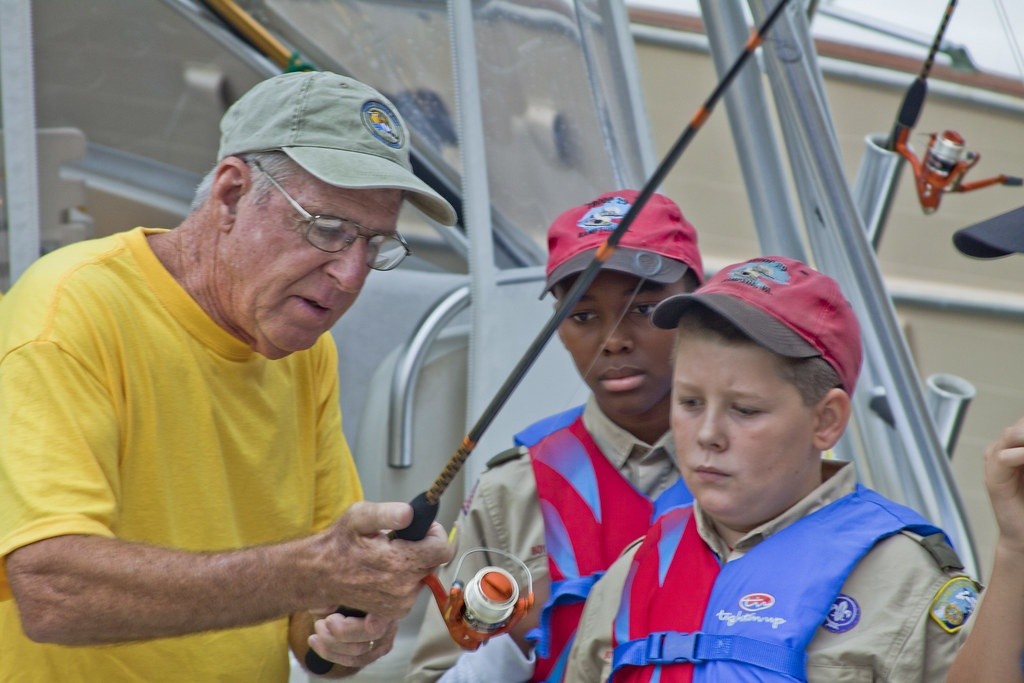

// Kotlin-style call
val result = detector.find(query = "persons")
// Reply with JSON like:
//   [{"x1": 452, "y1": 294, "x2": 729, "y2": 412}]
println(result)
[
  {"x1": 396, "y1": 189, "x2": 706, "y2": 683},
  {"x1": 942, "y1": 417, "x2": 1024, "y2": 683},
  {"x1": 0, "y1": 72, "x2": 452, "y2": 681},
  {"x1": 556, "y1": 254, "x2": 982, "y2": 683}
]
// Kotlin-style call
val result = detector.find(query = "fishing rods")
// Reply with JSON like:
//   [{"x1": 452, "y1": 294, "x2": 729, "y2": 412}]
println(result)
[
  {"x1": 298, "y1": 0, "x2": 798, "y2": 678},
  {"x1": 873, "y1": 0, "x2": 1024, "y2": 218},
  {"x1": 192, "y1": 0, "x2": 541, "y2": 270}
]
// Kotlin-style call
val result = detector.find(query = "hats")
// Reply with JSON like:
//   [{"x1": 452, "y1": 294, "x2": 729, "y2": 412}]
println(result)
[
  {"x1": 647, "y1": 255, "x2": 864, "y2": 403},
  {"x1": 539, "y1": 187, "x2": 709, "y2": 301},
  {"x1": 213, "y1": 67, "x2": 461, "y2": 227}
]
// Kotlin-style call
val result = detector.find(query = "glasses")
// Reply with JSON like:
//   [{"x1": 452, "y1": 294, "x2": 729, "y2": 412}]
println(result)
[{"x1": 246, "y1": 159, "x2": 413, "y2": 273}]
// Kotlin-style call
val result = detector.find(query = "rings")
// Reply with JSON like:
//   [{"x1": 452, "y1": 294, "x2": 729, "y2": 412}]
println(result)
[{"x1": 368, "y1": 640, "x2": 374, "y2": 652}]
